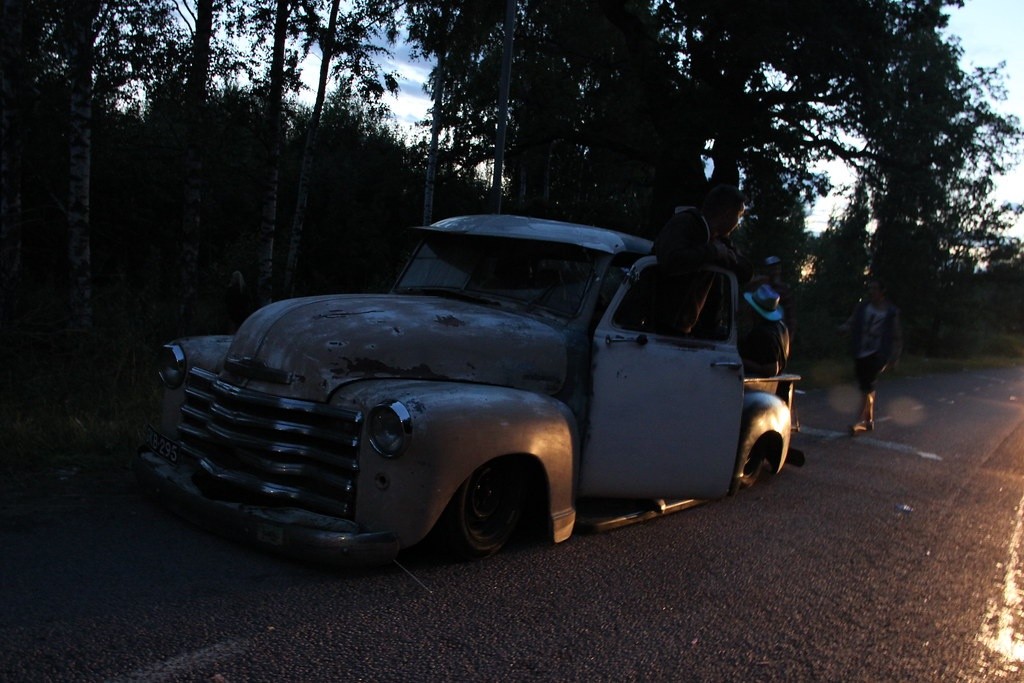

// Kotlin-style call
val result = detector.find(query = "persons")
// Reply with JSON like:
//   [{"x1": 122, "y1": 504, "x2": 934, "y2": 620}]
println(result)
[
  {"x1": 836, "y1": 280, "x2": 903, "y2": 436},
  {"x1": 621, "y1": 184, "x2": 751, "y2": 338},
  {"x1": 738, "y1": 269, "x2": 797, "y2": 379}
]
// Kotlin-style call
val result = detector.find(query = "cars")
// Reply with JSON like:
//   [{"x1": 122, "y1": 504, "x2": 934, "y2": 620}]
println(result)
[{"x1": 129, "y1": 214, "x2": 804, "y2": 566}]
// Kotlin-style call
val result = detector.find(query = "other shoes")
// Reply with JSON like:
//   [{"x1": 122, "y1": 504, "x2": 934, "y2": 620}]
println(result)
[{"x1": 848, "y1": 422, "x2": 868, "y2": 431}]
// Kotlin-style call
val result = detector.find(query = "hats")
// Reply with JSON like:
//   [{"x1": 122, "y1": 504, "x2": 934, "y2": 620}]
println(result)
[{"x1": 743, "y1": 283, "x2": 784, "y2": 320}]
